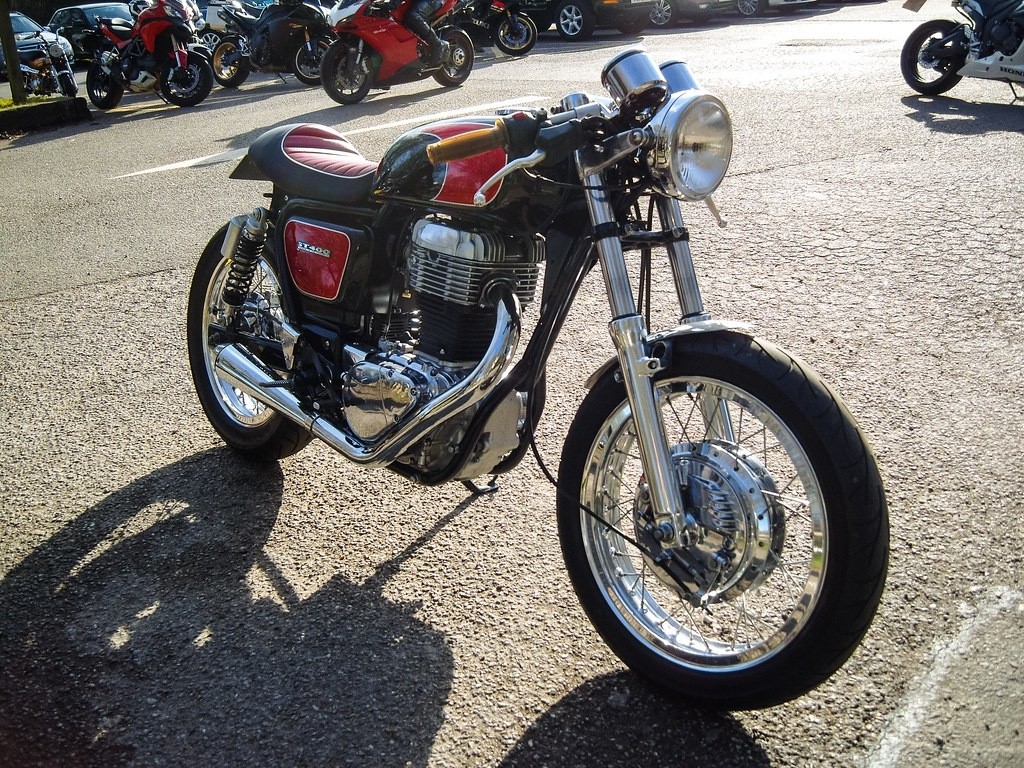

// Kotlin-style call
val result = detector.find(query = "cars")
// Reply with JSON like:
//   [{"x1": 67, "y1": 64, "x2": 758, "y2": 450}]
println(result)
[
  {"x1": 46, "y1": 1, "x2": 137, "y2": 61},
  {"x1": 0, "y1": 10, "x2": 77, "y2": 81}
]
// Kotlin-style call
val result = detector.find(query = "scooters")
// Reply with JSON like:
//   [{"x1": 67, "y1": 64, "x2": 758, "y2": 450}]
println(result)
[{"x1": 900, "y1": 0, "x2": 1024, "y2": 99}]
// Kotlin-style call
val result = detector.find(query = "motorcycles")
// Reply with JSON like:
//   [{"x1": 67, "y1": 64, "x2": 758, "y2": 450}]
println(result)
[
  {"x1": 185, "y1": 49, "x2": 891, "y2": 713},
  {"x1": 190, "y1": 0, "x2": 813, "y2": 58},
  {"x1": 3, "y1": 21, "x2": 80, "y2": 99},
  {"x1": 75, "y1": 0, "x2": 215, "y2": 110},
  {"x1": 319, "y1": 0, "x2": 476, "y2": 106},
  {"x1": 210, "y1": 0, "x2": 338, "y2": 90}
]
